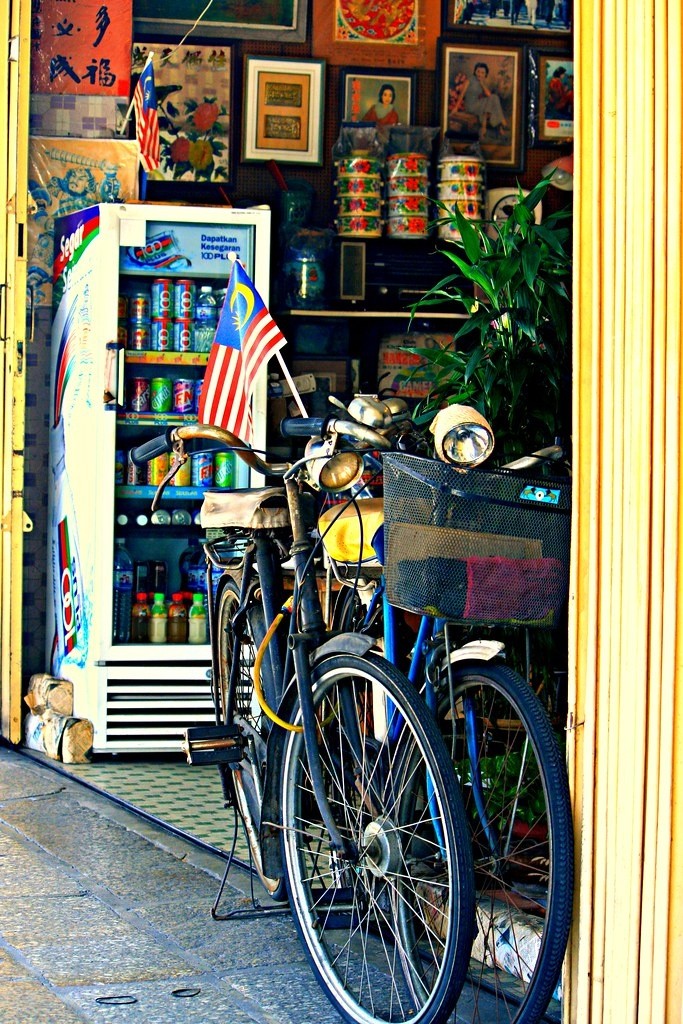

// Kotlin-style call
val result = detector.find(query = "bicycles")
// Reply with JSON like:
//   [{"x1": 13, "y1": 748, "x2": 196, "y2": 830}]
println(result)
[{"x1": 128, "y1": 392, "x2": 579, "y2": 1024}]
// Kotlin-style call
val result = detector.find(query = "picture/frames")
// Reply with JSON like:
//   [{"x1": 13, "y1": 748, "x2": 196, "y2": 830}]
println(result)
[{"x1": 133, "y1": 0, "x2": 574, "y2": 184}]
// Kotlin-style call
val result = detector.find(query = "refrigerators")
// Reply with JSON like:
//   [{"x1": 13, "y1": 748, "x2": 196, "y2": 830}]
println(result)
[{"x1": 44, "y1": 202, "x2": 271, "y2": 759}]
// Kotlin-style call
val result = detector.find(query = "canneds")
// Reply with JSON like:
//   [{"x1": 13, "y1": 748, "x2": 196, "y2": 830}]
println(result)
[
  {"x1": 132, "y1": 559, "x2": 167, "y2": 596},
  {"x1": 149, "y1": 508, "x2": 201, "y2": 526},
  {"x1": 116, "y1": 447, "x2": 233, "y2": 487},
  {"x1": 120, "y1": 278, "x2": 196, "y2": 352},
  {"x1": 128, "y1": 376, "x2": 203, "y2": 413}
]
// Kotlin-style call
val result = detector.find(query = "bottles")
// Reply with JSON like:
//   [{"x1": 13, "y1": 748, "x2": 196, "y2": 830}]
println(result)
[
  {"x1": 116, "y1": 505, "x2": 202, "y2": 526},
  {"x1": 112, "y1": 537, "x2": 223, "y2": 643},
  {"x1": 194, "y1": 286, "x2": 228, "y2": 354}
]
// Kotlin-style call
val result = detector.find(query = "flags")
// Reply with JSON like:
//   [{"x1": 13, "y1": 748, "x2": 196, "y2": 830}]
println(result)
[
  {"x1": 133, "y1": 59, "x2": 161, "y2": 171},
  {"x1": 198, "y1": 258, "x2": 289, "y2": 452}
]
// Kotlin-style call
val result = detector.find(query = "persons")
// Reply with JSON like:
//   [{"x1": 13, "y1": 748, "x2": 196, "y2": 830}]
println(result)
[
  {"x1": 461, "y1": 0, "x2": 573, "y2": 29},
  {"x1": 360, "y1": 84, "x2": 397, "y2": 131},
  {"x1": 548, "y1": 67, "x2": 574, "y2": 121},
  {"x1": 451, "y1": 63, "x2": 510, "y2": 142}
]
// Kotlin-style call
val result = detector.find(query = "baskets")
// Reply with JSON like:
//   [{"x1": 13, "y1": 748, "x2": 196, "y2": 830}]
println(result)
[{"x1": 377, "y1": 452, "x2": 568, "y2": 625}]
